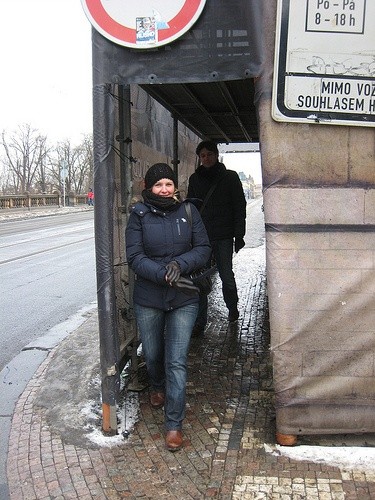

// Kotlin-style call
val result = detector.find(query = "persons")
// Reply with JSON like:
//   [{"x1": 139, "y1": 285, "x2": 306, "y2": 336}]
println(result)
[
  {"x1": 88, "y1": 187, "x2": 94, "y2": 207},
  {"x1": 124, "y1": 162, "x2": 213, "y2": 452},
  {"x1": 185, "y1": 139, "x2": 247, "y2": 340}
]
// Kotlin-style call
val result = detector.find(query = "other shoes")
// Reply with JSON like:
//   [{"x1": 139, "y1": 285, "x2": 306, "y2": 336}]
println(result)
[
  {"x1": 226, "y1": 307, "x2": 239, "y2": 321},
  {"x1": 191, "y1": 328, "x2": 205, "y2": 337}
]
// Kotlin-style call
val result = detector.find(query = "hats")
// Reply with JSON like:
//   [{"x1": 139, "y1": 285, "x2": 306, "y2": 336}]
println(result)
[{"x1": 144, "y1": 163, "x2": 177, "y2": 190}]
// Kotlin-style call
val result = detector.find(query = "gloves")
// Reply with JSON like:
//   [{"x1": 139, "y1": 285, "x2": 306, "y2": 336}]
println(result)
[
  {"x1": 172, "y1": 276, "x2": 199, "y2": 297},
  {"x1": 165, "y1": 261, "x2": 181, "y2": 283}
]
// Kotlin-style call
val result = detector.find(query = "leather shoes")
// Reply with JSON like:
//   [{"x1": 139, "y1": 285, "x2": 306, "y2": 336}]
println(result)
[
  {"x1": 149, "y1": 387, "x2": 165, "y2": 409},
  {"x1": 166, "y1": 430, "x2": 183, "y2": 451}
]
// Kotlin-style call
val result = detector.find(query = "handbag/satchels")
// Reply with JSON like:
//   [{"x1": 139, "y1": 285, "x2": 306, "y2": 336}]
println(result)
[{"x1": 184, "y1": 202, "x2": 217, "y2": 282}]
